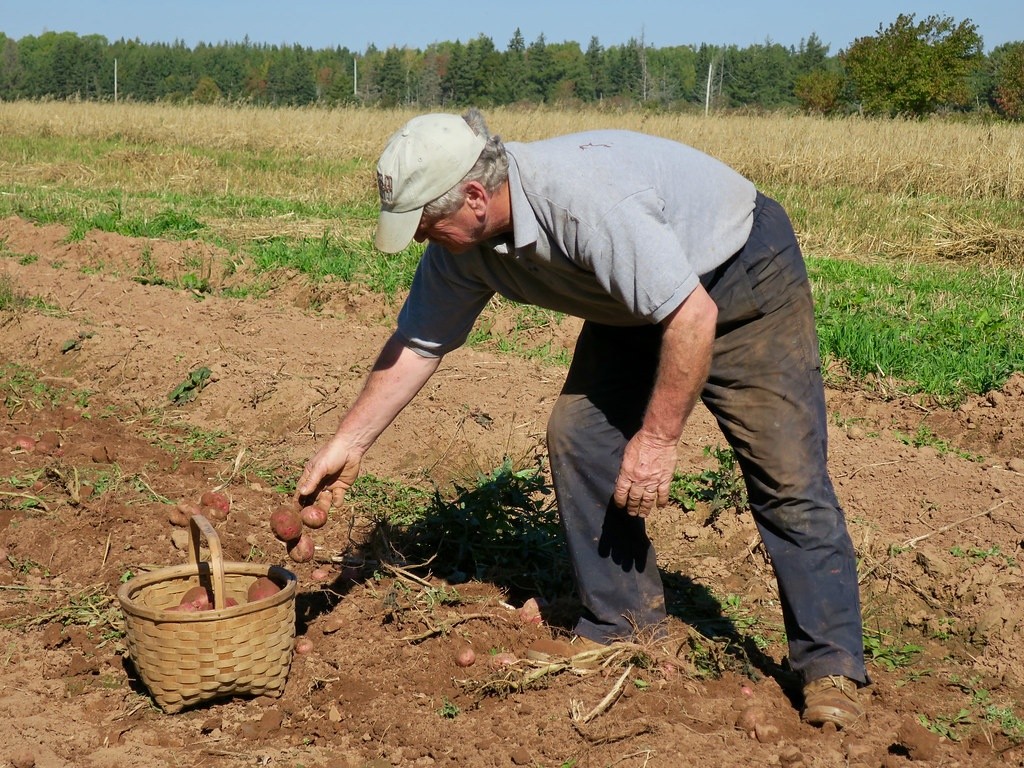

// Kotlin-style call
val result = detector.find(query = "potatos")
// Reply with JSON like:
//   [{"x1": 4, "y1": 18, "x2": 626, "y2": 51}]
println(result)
[{"x1": 9, "y1": 435, "x2": 754, "y2": 698}]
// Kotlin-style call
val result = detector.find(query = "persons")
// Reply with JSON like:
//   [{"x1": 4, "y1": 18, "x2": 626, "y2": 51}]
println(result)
[{"x1": 295, "y1": 112, "x2": 872, "y2": 727}]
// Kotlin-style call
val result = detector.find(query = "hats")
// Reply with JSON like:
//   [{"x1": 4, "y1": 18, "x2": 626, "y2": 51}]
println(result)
[{"x1": 372, "y1": 112, "x2": 489, "y2": 254}]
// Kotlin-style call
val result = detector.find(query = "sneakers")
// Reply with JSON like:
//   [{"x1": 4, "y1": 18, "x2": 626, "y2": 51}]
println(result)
[
  {"x1": 802, "y1": 673, "x2": 865, "y2": 730},
  {"x1": 528, "y1": 632, "x2": 607, "y2": 663}
]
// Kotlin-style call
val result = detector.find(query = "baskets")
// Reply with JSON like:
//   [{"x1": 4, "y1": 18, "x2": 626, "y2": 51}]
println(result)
[{"x1": 117, "y1": 513, "x2": 298, "y2": 716}]
